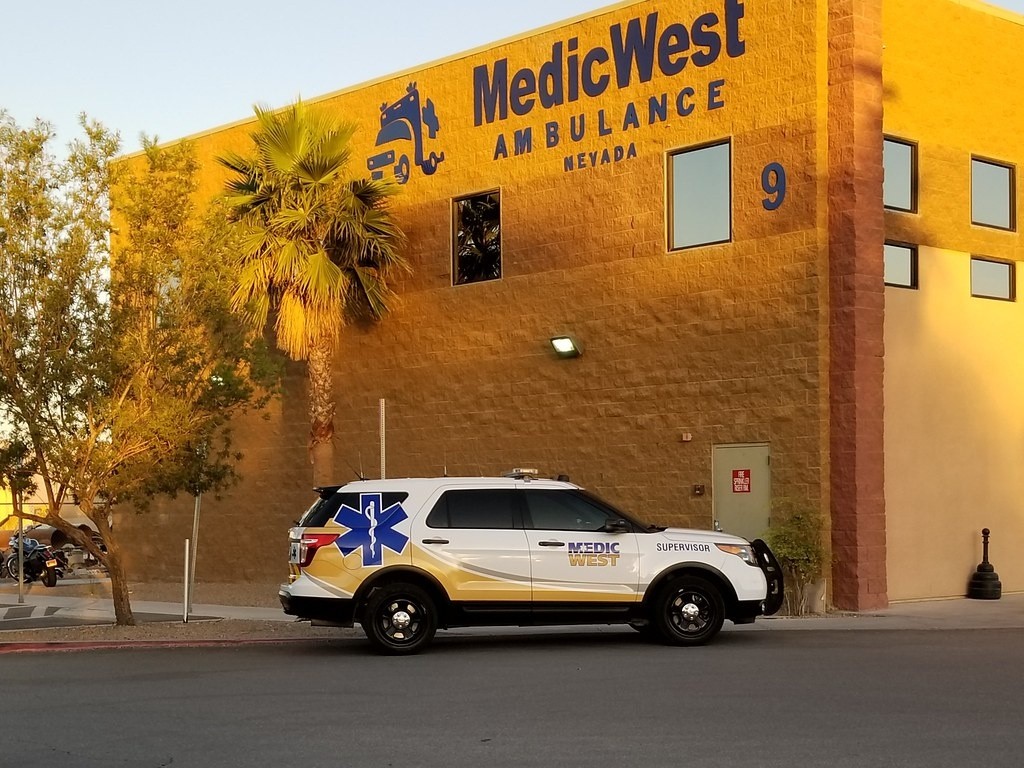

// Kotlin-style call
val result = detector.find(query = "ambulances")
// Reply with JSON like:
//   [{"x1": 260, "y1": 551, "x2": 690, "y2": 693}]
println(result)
[{"x1": 278, "y1": 468, "x2": 784, "y2": 655}]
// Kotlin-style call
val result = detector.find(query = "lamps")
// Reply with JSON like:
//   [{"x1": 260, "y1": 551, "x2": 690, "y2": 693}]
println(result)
[{"x1": 550, "y1": 334, "x2": 584, "y2": 359}]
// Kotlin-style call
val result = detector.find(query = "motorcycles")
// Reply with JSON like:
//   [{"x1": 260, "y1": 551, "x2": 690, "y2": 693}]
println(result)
[{"x1": 6, "y1": 524, "x2": 64, "y2": 587}]
